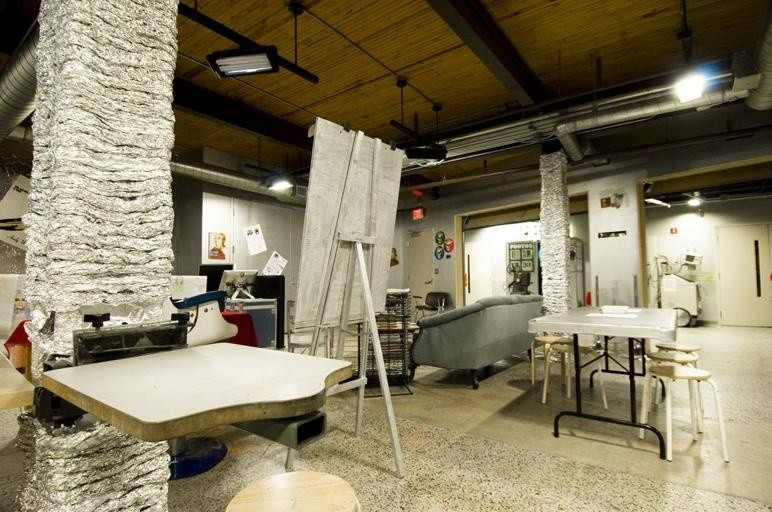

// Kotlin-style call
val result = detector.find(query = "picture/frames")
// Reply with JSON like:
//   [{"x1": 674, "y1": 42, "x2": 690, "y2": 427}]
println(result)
[{"x1": 507, "y1": 241, "x2": 536, "y2": 272}]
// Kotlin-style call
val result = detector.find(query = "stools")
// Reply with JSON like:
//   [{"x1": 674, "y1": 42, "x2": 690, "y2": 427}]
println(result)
[
  {"x1": 225, "y1": 471, "x2": 361, "y2": 512},
  {"x1": 528, "y1": 335, "x2": 729, "y2": 464}
]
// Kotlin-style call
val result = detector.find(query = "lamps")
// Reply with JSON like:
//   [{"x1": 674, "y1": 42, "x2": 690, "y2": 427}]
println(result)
[
  {"x1": 391, "y1": 77, "x2": 442, "y2": 148},
  {"x1": 403, "y1": 142, "x2": 449, "y2": 161},
  {"x1": 179, "y1": 0, "x2": 319, "y2": 87},
  {"x1": 207, "y1": 46, "x2": 279, "y2": 79}
]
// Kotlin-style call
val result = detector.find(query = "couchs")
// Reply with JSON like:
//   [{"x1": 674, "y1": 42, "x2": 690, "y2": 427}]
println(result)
[{"x1": 410, "y1": 294, "x2": 543, "y2": 390}]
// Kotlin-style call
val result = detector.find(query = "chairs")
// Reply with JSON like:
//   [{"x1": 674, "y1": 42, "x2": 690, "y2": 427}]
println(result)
[
  {"x1": 286, "y1": 300, "x2": 333, "y2": 358},
  {"x1": 414, "y1": 292, "x2": 448, "y2": 323}
]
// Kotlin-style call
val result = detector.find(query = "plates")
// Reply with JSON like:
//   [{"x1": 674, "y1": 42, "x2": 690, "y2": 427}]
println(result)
[{"x1": 600, "y1": 305, "x2": 629, "y2": 314}]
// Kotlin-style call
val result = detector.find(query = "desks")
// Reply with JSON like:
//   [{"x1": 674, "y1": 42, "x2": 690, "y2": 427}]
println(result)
[
  {"x1": 43, "y1": 342, "x2": 354, "y2": 481},
  {"x1": 525, "y1": 303, "x2": 684, "y2": 460}
]
